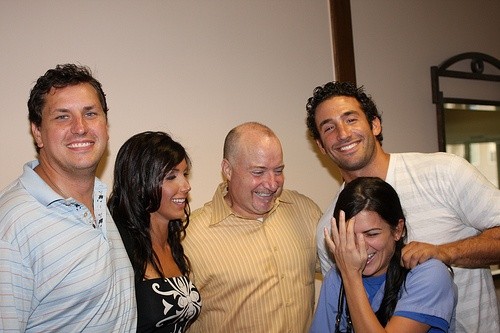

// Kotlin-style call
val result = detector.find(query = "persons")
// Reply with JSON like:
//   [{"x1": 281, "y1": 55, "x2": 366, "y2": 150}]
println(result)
[
  {"x1": 0, "y1": 63, "x2": 138, "y2": 333},
  {"x1": 308, "y1": 176, "x2": 459, "y2": 333},
  {"x1": 106, "y1": 131, "x2": 203, "y2": 333},
  {"x1": 178, "y1": 122, "x2": 324, "y2": 333},
  {"x1": 305, "y1": 81, "x2": 500, "y2": 333}
]
